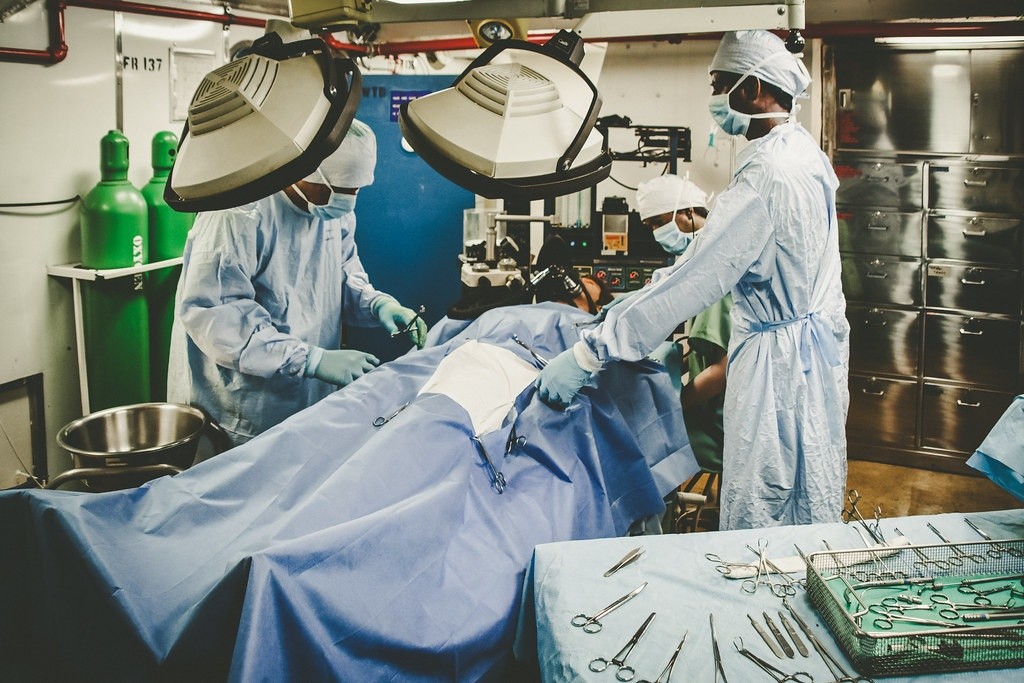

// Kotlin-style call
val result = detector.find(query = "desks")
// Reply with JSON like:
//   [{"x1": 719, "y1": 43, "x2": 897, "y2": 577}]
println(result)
[{"x1": 513, "y1": 507, "x2": 1023, "y2": 683}]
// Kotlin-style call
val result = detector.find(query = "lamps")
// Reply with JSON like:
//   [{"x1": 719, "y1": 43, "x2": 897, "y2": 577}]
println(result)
[
  {"x1": 398, "y1": 29, "x2": 614, "y2": 200},
  {"x1": 466, "y1": 16, "x2": 530, "y2": 47},
  {"x1": 163, "y1": 34, "x2": 364, "y2": 209}
]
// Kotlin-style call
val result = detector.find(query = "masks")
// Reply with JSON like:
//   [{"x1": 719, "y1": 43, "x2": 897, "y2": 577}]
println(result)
[
  {"x1": 292, "y1": 168, "x2": 356, "y2": 222},
  {"x1": 707, "y1": 94, "x2": 750, "y2": 138},
  {"x1": 652, "y1": 222, "x2": 703, "y2": 256}
]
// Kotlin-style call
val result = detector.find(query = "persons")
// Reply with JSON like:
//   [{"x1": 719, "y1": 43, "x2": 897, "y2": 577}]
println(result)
[
  {"x1": 167, "y1": 119, "x2": 427, "y2": 465},
  {"x1": 534, "y1": 31, "x2": 850, "y2": 530},
  {"x1": 556, "y1": 273, "x2": 616, "y2": 315},
  {"x1": 637, "y1": 176, "x2": 731, "y2": 535}
]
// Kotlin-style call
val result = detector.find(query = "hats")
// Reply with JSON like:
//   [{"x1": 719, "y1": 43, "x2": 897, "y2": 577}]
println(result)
[
  {"x1": 301, "y1": 118, "x2": 377, "y2": 188},
  {"x1": 638, "y1": 174, "x2": 709, "y2": 221},
  {"x1": 707, "y1": 30, "x2": 811, "y2": 97}
]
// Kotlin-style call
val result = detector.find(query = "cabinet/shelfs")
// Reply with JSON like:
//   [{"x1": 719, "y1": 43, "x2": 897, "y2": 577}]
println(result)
[{"x1": 822, "y1": 36, "x2": 1023, "y2": 477}]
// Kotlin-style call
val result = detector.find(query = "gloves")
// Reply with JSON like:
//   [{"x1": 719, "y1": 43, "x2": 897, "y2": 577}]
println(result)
[
  {"x1": 534, "y1": 341, "x2": 603, "y2": 408},
  {"x1": 370, "y1": 297, "x2": 427, "y2": 349},
  {"x1": 308, "y1": 347, "x2": 380, "y2": 387}
]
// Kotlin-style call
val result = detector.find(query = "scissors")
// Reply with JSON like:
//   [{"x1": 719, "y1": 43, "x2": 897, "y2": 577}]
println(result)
[
  {"x1": 472, "y1": 436, "x2": 506, "y2": 494},
  {"x1": 745, "y1": 543, "x2": 808, "y2": 596},
  {"x1": 822, "y1": 539, "x2": 869, "y2": 582},
  {"x1": 571, "y1": 582, "x2": 648, "y2": 634},
  {"x1": 868, "y1": 584, "x2": 1015, "y2": 630},
  {"x1": 794, "y1": 544, "x2": 808, "y2": 563},
  {"x1": 507, "y1": 423, "x2": 526, "y2": 454},
  {"x1": 705, "y1": 553, "x2": 753, "y2": 574},
  {"x1": 841, "y1": 489, "x2": 987, "y2": 581},
  {"x1": 373, "y1": 401, "x2": 410, "y2": 426},
  {"x1": 963, "y1": 518, "x2": 1023, "y2": 559},
  {"x1": 636, "y1": 632, "x2": 688, "y2": 683},
  {"x1": 390, "y1": 305, "x2": 425, "y2": 339},
  {"x1": 742, "y1": 538, "x2": 788, "y2": 597},
  {"x1": 732, "y1": 637, "x2": 815, "y2": 683},
  {"x1": 782, "y1": 598, "x2": 875, "y2": 683},
  {"x1": 710, "y1": 613, "x2": 728, "y2": 683},
  {"x1": 589, "y1": 613, "x2": 656, "y2": 682}
]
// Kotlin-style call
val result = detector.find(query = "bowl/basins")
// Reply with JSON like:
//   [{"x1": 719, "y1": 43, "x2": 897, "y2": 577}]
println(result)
[{"x1": 54, "y1": 402, "x2": 208, "y2": 492}]
